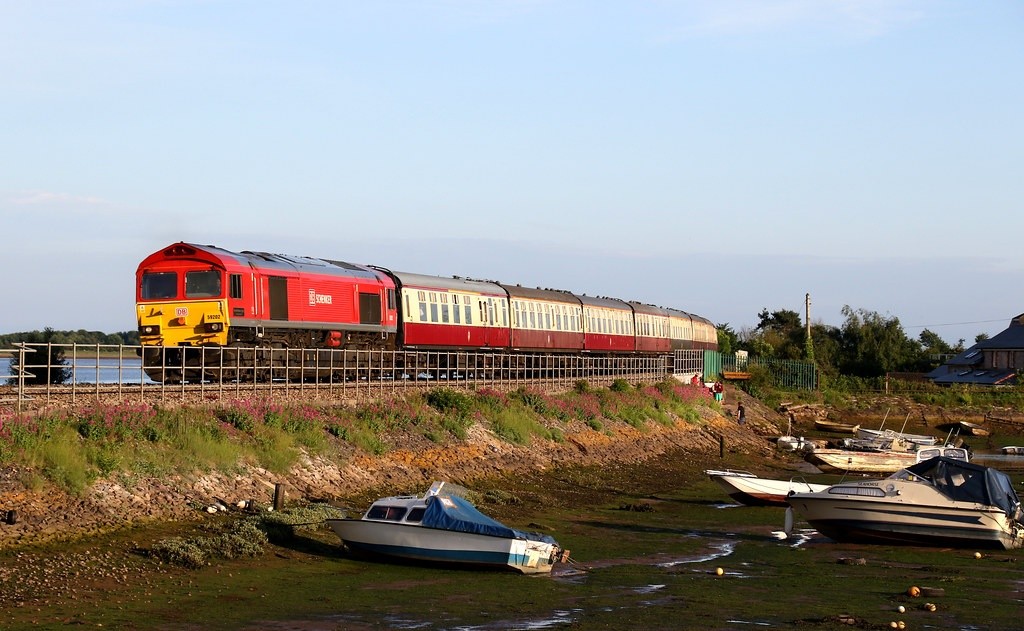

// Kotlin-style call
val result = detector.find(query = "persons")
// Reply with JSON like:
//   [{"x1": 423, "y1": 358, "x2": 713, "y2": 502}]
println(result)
[
  {"x1": 712, "y1": 381, "x2": 724, "y2": 403},
  {"x1": 213, "y1": 277, "x2": 220, "y2": 294},
  {"x1": 737, "y1": 401, "x2": 745, "y2": 426},
  {"x1": 692, "y1": 375, "x2": 698, "y2": 386}
]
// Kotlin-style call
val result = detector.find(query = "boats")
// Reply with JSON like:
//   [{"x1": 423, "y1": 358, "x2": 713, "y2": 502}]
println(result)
[
  {"x1": 815, "y1": 420, "x2": 860, "y2": 434},
  {"x1": 703, "y1": 469, "x2": 832, "y2": 505},
  {"x1": 960, "y1": 420, "x2": 992, "y2": 437},
  {"x1": 784, "y1": 455, "x2": 1024, "y2": 552},
  {"x1": 324, "y1": 480, "x2": 571, "y2": 576},
  {"x1": 777, "y1": 417, "x2": 1024, "y2": 475}
]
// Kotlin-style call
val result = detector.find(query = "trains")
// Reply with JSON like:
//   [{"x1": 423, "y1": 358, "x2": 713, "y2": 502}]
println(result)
[{"x1": 131, "y1": 241, "x2": 721, "y2": 386}]
